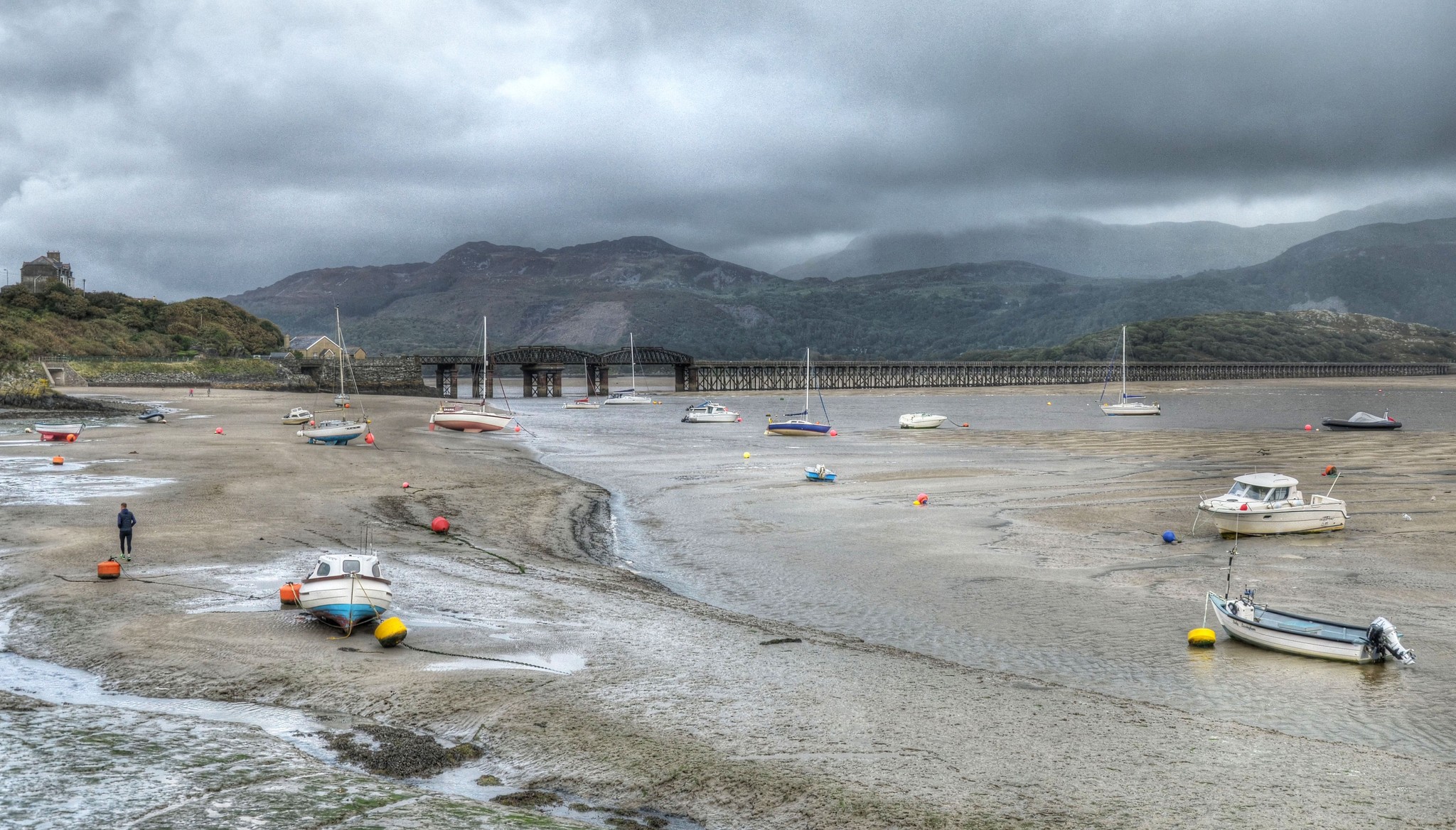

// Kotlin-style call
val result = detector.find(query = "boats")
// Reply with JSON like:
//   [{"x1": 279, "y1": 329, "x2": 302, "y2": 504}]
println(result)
[
  {"x1": 603, "y1": 332, "x2": 652, "y2": 404},
  {"x1": 680, "y1": 403, "x2": 740, "y2": 422},
  {"x1": 432, "y1": 314, "x2": 513, "y2": 433},
  {"x1": 297, "y1": 522, "x2": 394, "y2": 636},
  {"x1": 898, "y1": 411, "x2": 948, "y2": 428},
  {"x1": 764, "y1": 347, "x2": 832, "y2": 436},
  {"x1": 1194, "y1": 465, "x2": 1352, "y2": 534},
  {"x1": 138, "y1": 406, "x2": 164, "y2": 423},
  {"x1": 1207, "y1": 545, "x2": 1417, "y2": 665},
  {"x1": 300, "y1": 296, "x2": 370, "y2": 446},
  {"x1": 804, "y1": 464, "x2": 836, "y2": 482},
  {"x1": 1094, "y1": 324, "x2": 1162, "y2": 416},
  {"x1": 34, "y1": 421, "x2": 86, "y2": 443},
  {"x1": 1321, "y1": 407, "x2": 1403, "y2": 430},
  {"x1": 562, "y1": 358, "x2": 600, "y2": 408},
  {"x1": 686, "y1": 398, "x2": 727, "y2": 412},
  {"x1": 281, "y1": 407, "x2": 312, "y2": 425}
]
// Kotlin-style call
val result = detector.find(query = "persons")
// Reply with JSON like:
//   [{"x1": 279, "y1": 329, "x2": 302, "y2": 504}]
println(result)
[
  {"x1": 207, "y1": 389, "x2": 210, "y2": 397},
  {"x1": 117, "y1": 503, "x2": 136, "y2": 560},
  {"x1": 189, "y1": 388, "x2": 193, "y2": 396}
]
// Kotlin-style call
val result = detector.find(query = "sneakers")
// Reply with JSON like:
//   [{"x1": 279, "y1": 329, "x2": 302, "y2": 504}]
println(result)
[
  {"x1": 126, "y1": 556, "x2": 131, "y2": 560},
  {"x1": 120, "y1": 553, "x2": 126, "y2": 559}
]
